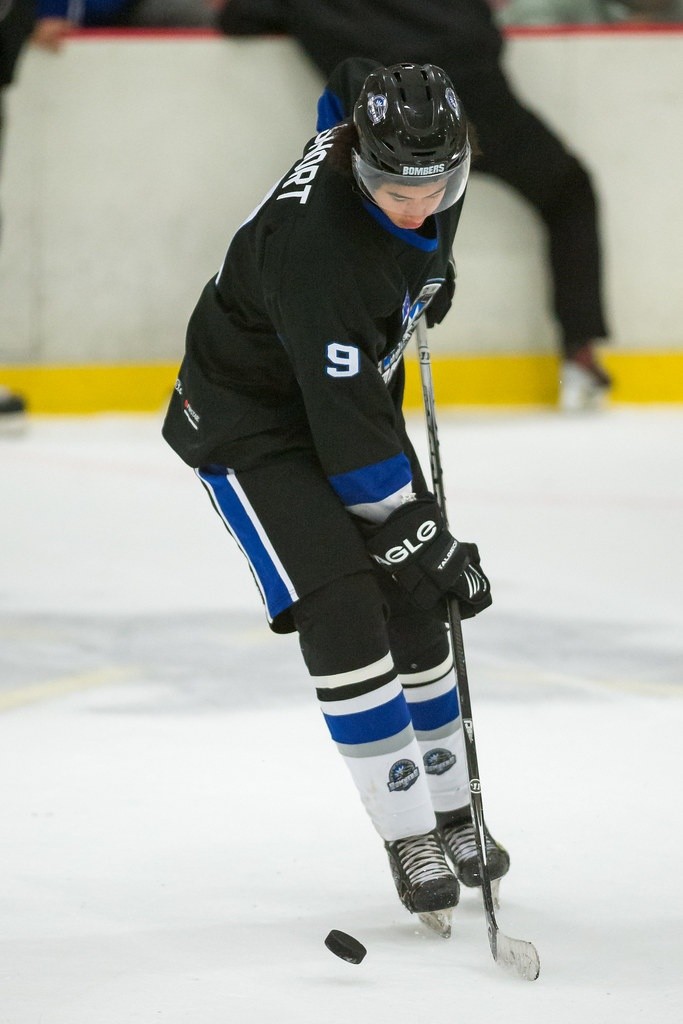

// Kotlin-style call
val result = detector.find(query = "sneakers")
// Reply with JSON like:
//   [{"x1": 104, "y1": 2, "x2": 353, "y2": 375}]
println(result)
[
  {"x1": 554, "y1": 336, "x2": 614, "y2": 414},
  {"x1": 385, "y1": 831, "x2": 462, "y2": 939},
  {"x1": 437, "y1": 818, "x2": 510, "y2": 912},
  {"x1": 0, "y1": 392, "x2": 30, "y2": 442}
]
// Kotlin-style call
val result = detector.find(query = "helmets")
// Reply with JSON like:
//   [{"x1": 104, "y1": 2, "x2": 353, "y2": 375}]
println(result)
[{"x1": 352, "y1": 61, "x2": 466, "y2": 181}]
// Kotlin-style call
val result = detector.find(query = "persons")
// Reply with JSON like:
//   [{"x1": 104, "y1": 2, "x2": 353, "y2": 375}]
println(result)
[
  {"x1": 215, "y1": 0, "x2": 614, "y2": 406},
  {"x1": 161, "y1": 59, "x2": 510, "y2": 937}
]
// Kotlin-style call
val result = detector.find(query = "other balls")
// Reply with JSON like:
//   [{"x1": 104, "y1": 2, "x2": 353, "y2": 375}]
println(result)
[{"x1": 324, "y1": 929, "x2": 367, "y2": 965}]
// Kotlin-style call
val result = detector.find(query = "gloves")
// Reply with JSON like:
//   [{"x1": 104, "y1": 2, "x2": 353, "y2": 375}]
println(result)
[
  {"x1": 360, "y1": 493, "x2": 494, "y2": 623},
  {"x1": 410, "y1": 251, "x2": 457, "y2": 329}
]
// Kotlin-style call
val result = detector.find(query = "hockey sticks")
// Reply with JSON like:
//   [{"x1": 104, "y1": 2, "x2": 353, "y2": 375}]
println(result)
[{"x1": 405, "y1": 313, "x2": 544, "y2": 984}]
